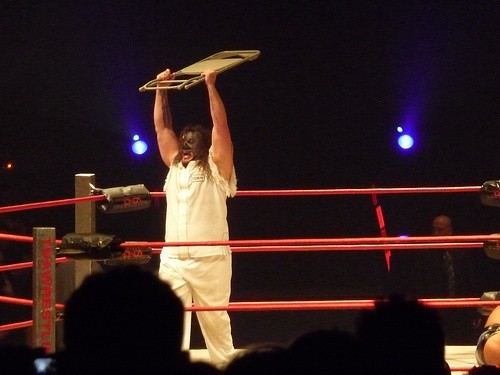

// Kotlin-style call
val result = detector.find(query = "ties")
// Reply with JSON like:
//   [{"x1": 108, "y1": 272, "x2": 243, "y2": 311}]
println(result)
[{"x1": 443, "y1": 251, "x2": 456, "y2": 298}]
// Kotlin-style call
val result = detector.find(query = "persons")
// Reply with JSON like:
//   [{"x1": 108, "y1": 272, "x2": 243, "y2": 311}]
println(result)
[
  {"x1": 475, "y1": 306, "x2": 500, "y2": 368},
  {"x1": 419, "y1": 215, "x2": 480, "y2": 344},
  {"x1": 0, "y1": 265, "x2": 450, "y2": 375},
  {"x1": 154, "y1": 69, "x2": 236, "y2": 370}
]
